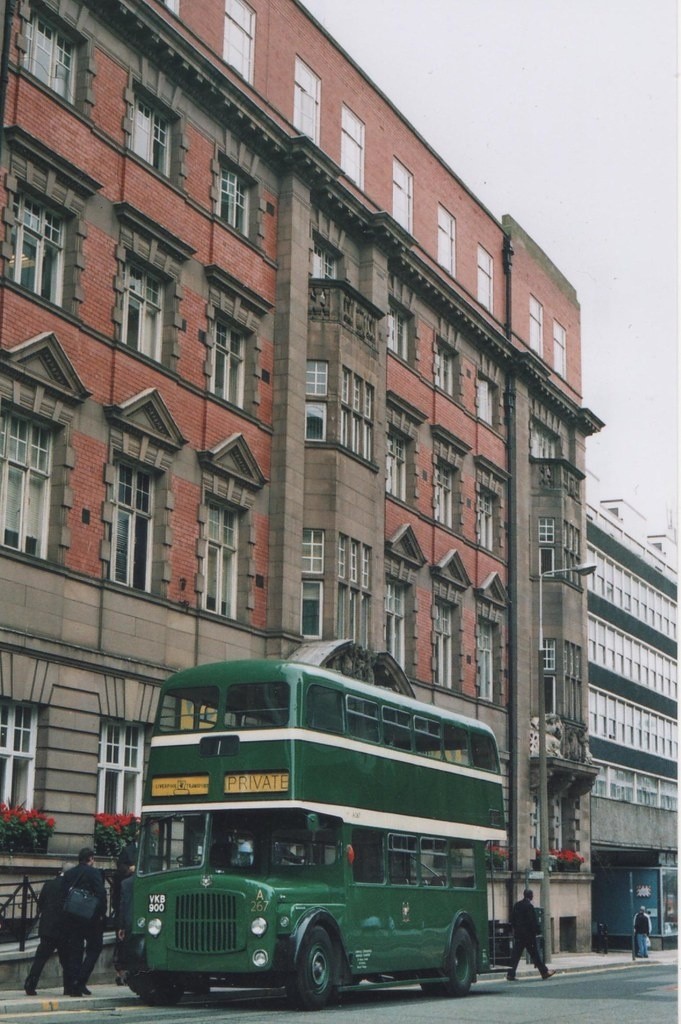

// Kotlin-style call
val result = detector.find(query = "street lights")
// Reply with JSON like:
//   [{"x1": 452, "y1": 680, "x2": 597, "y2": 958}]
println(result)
[{"x1": 539, "y1": 559, "x2": 598, "y2": 967}]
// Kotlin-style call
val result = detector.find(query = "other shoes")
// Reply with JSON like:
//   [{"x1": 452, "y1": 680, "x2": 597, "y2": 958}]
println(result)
[
  {"x1": 82, "y1": 987, "x2": 91, "y2": 995},
  {"x1": 123, "y1": 978, "x2": 129, "y2": 986},
  {"x1": 116, "y1": 977, "x2": 124, "y2": 986},
  {"x1": 72, "y1": 980, "x2": 83, "y2": 997},
  {"x1": 24, "y1": 980, "x2": 37, "y2": 995},
  {"x1": 64, "y1": 985, "x2": 73, "y2": 995}
]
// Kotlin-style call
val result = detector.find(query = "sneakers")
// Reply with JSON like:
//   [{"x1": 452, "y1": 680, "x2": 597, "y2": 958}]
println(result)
[
  {"x1": 541, "y1": 970, "x2": 556, "y2": 979},
  {"x1": 507, "y1": 976, "x2": 519, "y2": 981}
]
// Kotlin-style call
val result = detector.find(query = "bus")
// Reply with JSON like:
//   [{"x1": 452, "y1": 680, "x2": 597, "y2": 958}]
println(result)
[{"x1": 127, "y1": 660, "x2": 505, "y2": 1006}]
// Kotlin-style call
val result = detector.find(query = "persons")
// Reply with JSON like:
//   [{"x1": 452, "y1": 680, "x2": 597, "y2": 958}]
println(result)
[
  {"x1": 633, "y1": 905, "x2": 652, "y2": 958},
  {"x1": 109, "y1": 829, "x2": 156, "y2": 985},
  {"x1": 62, "y1": 847, "x2": 107, "y2": 996},
  {"x1": 118, "y1": 865, "x2": 136, "y2": 991},
  {"x1": 505, "y1": 889, "x2": 556, "y2": 980},
  {"x1": 25, "y1": 861, "x2": 77, "y2": 997}
]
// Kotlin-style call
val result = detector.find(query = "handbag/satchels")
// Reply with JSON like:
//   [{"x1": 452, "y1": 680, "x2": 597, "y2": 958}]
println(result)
[
  {"x1": 646, "y1": 936, "x2": 651, "y2": 948},
  {"x1": 62, "y1": 888, "x2": 101, "y2": 919}
]
